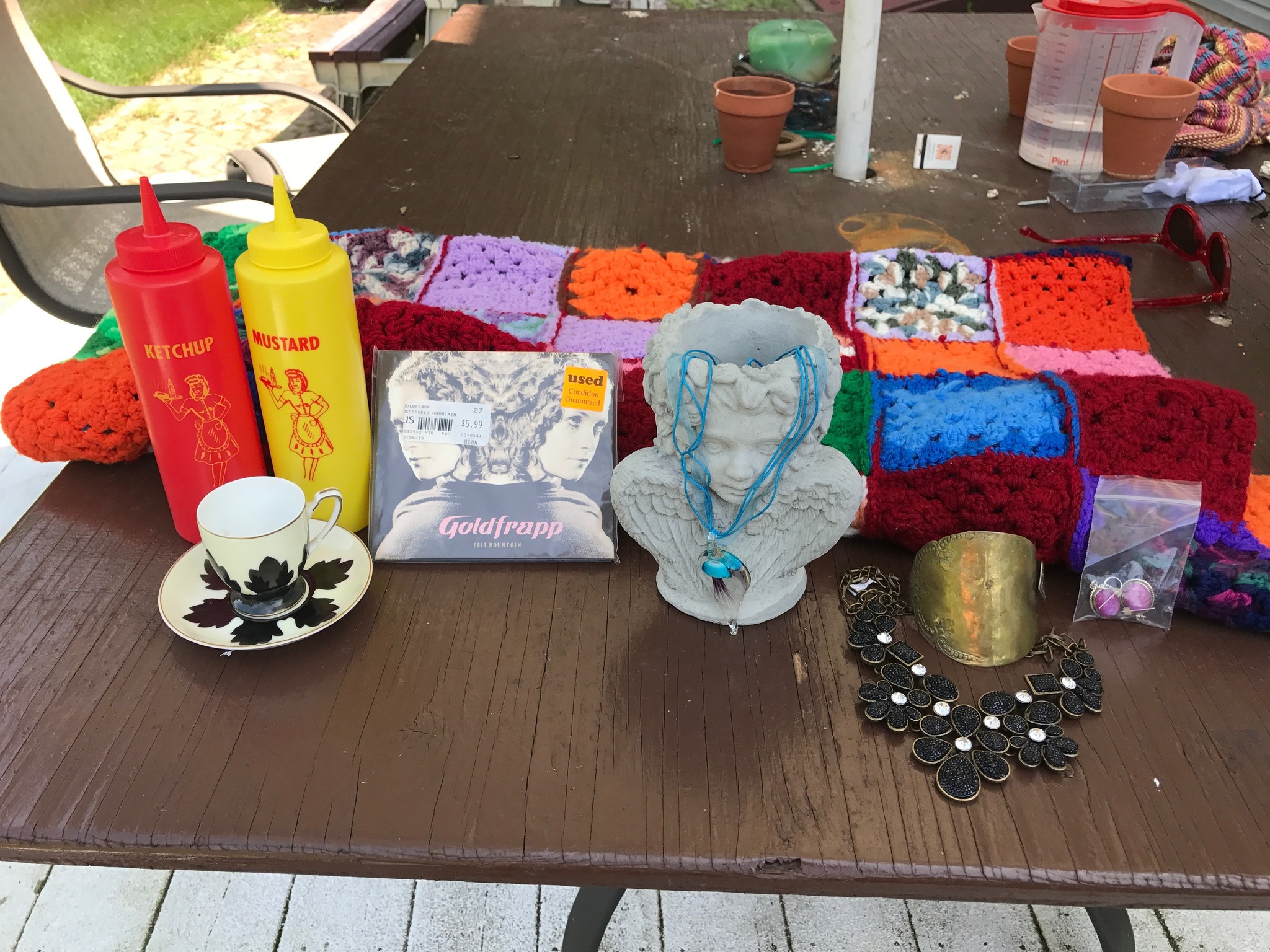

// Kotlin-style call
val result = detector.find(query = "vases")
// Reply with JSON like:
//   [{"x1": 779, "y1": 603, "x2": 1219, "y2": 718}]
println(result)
[
  {"x1": 1099, "y1": 73, "x2": 1201, "y2": 181},
  {"x1": 713, "y1": 76, "x2": 796, "y2": 173},
  {"x1": 1006, "y1": 35, "x2": 1038, "y2": 118}
]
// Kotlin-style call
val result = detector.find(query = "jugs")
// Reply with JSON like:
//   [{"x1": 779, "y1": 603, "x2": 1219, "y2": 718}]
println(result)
[{"x1": 1018, "y1": 0, "x2": 1207, "y2": 173}]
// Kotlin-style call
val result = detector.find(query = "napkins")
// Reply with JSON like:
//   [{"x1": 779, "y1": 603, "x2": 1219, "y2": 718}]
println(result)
[{"x1": 1143, "y1": 161, "x2": 1266, "y2": 204}]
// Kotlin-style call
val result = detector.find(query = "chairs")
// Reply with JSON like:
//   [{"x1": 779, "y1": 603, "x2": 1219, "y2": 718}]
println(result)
[{"x1": 0, "y1": 0, "x2": 357, "y2": 328}]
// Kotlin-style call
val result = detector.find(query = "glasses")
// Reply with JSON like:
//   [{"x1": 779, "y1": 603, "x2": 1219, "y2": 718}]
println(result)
[{"x1": 1018, "y1": 203, "x2": 1233, "y2": 307}]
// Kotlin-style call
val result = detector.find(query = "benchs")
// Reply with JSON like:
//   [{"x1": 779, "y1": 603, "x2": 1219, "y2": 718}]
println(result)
[{"x1": 310, "y1": 0, "x2": 426, "y2": 131}]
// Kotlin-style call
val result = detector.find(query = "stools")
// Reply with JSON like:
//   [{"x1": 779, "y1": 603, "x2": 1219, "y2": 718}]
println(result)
[{"x1": 226, "y1": 133, "x2": 350, "y2": 196}]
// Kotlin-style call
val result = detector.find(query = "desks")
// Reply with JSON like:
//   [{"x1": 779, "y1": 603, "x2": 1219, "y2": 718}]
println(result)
[{"x1": 0, "y1": 5, "x2": 1270, "y2": 952}]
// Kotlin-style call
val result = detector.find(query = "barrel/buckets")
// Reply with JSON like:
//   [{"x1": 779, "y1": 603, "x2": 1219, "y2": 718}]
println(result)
[
  {"x1": 1099, "y1": 73, "x2": 1201, "y2": 180},
  {"x1": 1005, "y1": 35, "x2": 1079, "y2": 118},
  {"x1": 713, "y1": 75, "x2": 795, "y2": 173}
]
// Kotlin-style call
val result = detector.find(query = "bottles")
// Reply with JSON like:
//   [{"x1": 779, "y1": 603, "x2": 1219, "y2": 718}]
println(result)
[
  {"x1": 236, "y1": 174, "x2": 372, "y2": 534},
  {"x1": 107, "y1": 176, "x2": 267, "y2": 543}
]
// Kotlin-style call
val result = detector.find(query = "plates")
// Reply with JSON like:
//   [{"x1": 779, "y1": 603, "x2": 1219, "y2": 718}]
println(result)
[{"x1": 159, "y1": 517, "x2": 373, "y2": 650}]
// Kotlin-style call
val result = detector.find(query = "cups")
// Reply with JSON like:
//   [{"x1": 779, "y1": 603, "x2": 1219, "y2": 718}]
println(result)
[{"x1": 197, "y1": 475, "x2": 343, "y2": 621}]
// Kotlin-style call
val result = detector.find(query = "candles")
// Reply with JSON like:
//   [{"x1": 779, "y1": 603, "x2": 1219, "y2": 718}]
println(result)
[{"x1": 748, "y1": 19, "x2": 837, "y2": 83}]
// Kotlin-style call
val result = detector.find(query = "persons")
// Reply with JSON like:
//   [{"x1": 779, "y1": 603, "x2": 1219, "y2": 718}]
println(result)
[
  {"x1": 378, "y1": 350, "x2": 613, "y2": 559},
  {"x1": 610, "y1": 299, "x2": 866, "y2": 599}
]
